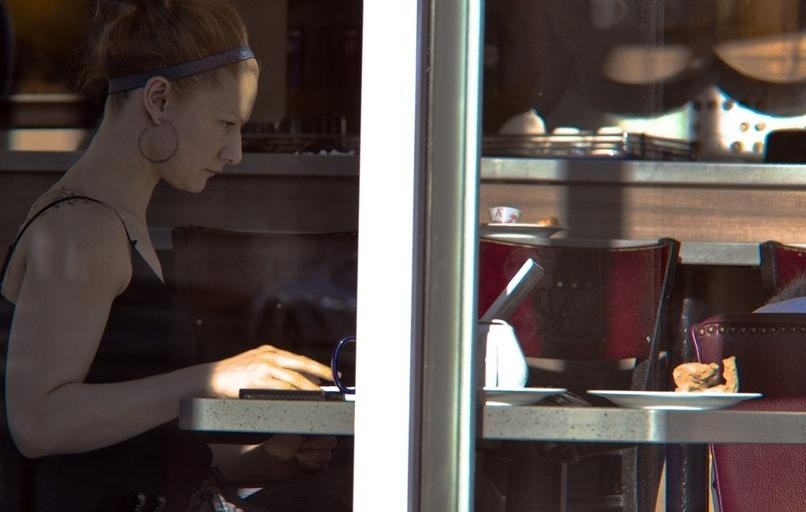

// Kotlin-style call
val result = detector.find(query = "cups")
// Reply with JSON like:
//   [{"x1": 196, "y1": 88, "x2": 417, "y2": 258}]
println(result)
[{"x1": 489, "y1": 207, "x2": 519, "y2": 223}]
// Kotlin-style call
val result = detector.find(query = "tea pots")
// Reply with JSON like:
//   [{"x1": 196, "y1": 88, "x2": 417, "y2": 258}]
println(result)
[{"x1": 473, "y1": 318, "x2": 529, "y2": 391}]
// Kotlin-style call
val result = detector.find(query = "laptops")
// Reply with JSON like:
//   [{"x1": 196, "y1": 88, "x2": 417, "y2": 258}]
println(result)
[{"x1": 316, "y1": 258, "x2": 545, "y2": 402}]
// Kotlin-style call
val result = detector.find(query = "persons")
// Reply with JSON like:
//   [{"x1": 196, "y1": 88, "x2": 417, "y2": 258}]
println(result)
[{"x1": 0, "y1": 1, "x2": 345, "y2": 511}]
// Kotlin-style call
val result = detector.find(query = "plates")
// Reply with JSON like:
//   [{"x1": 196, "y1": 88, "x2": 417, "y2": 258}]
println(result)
[
  {"x1": 479, "y1": 223, "x2": 571, "y2": 240},
  {"x1": 477, "y1": 388, "x2": 763, "y2": 410}
]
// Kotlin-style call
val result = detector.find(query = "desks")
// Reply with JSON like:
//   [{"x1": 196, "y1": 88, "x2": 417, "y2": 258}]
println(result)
[{"x1": 178, "y1": 371, "x2": 803, "y2": 505}]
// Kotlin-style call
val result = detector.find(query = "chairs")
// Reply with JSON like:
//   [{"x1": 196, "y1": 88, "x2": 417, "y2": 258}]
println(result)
[
  {"x1": 456, "y1": 228, "x2": 669, "y2": 394},
  {"x1": 677, "y1": 301, "x2": 804, "y2": 503}
]
